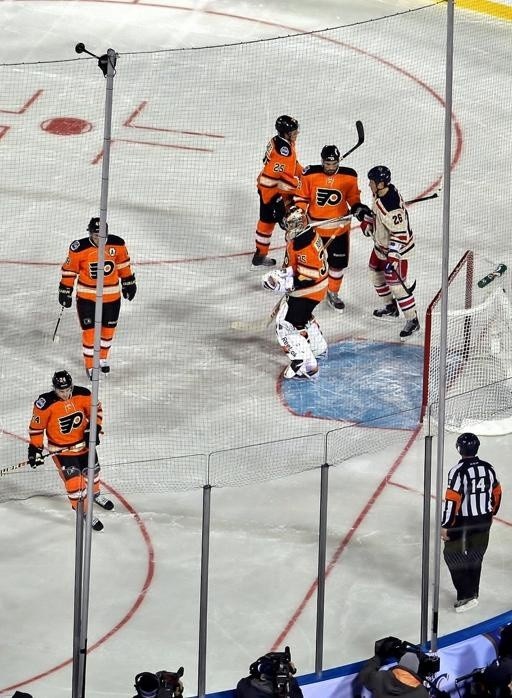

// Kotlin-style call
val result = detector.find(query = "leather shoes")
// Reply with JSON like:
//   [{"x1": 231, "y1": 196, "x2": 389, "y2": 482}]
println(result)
[
  {"x1": 121, "y1": 273, "x2": 136, "y2": 301},
  {"x1": 83, "y1": 423, "x2": 102, "y2": 448},
  {"x1": 351, "y1": 202, "x2": 371, "y2": 221},
  {"x1": 384, "y1": 252, "x2": 401, "y2": 275},
  {"x1": 360, "y1": 221, "x2": 373, "y2": 236},
  {"x1": 58, "y1": 282, "x2": 73, "y2": 308},
  {"x1": 28, "y1": 444, "x2": 43, "y2": 469}
]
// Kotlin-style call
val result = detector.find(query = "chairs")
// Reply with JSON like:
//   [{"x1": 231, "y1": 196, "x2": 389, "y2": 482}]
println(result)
[
  {"x1": 400, "y1": 317, "x2": 419, "y2": 336},
  {"x1": 82, "y1": 512, "x2": 103, "y2": 531},
  {"x1": 373, "y1": 299, "x2": 399, "y2": 317},
  {"x1": 252, "y1": 254, "x2": 276, "y2": 266},
  {"x1": 93, "y1": 495, "x2": 114, "y2": 510},
  {"x1": 99, "y1": 358, "x2": 110, "y2": 373},
  {"x1": 327, "y1": 291, "x2": 345, "y2": 309}
]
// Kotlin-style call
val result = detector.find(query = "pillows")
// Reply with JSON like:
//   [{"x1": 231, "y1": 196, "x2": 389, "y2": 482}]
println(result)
[
  {"x1": 86, "y1": 218, "x2": 108, "y2": 233},
  {"x1": 278, "y1": 205, "x2": 305, "y2": 231},
  {"x1": 368, "y1": 166, "x2": 391, "y2": 183},
  {"x1": 275, "y1": 116, "x2": 298, "y2": 132},
  {"x1": 321, "y1": 145, "x2": 340, "y2": 161},
  {"x1": 52, "y1": 371, "x2": 72, "y2": 389},
  {"x1": 456, "y1": 433, "x2": 480, "y2": 456}
]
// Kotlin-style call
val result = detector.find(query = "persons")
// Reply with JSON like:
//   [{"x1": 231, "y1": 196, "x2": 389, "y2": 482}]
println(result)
[
  {"x1": 360, "y1": 166, "x2": 420, "y2": 336},
  {"x1": 440, "y1": 433, "x2": 501, "y2": 612},
  {"x1": 132, "y1": 675, "x2": 184, "y2": 698},
  {"x1": 359, "y1": 639, "x2": 431, "y2": 698},
  {"x1": 293, "y1": 145, "x2": 371, "y2": 310},
  {"x1": 58, "y1": 216, "x2": 136, "y2": 381},
  {"x1": 236, "y1": 659, "x2": 304, "y2": 698},
  {"x1": 261, "y1": 206, "x2": 328, "y2": 379},
  {"x1": 29, "y1": 370, "x2": 115, "y2": 530},
  {"x1": 252, "y1": 115, "x2": 303, "y2": 267}
]
